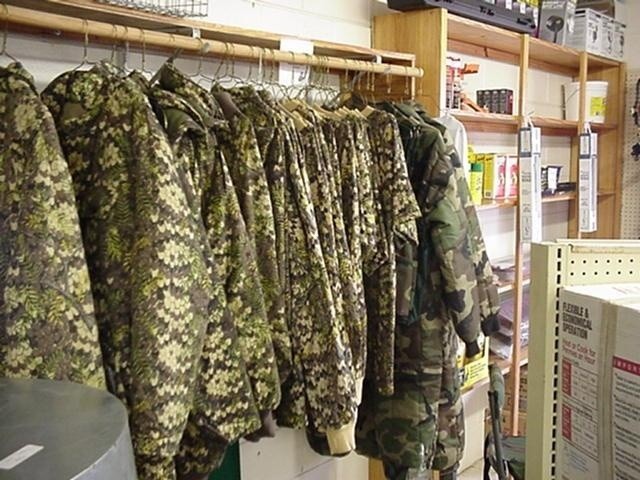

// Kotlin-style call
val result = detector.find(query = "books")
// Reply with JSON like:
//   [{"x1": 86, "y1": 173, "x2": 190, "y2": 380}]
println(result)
[{"x1": 485, "y1": 371, "x2": 528, "y2": 437}]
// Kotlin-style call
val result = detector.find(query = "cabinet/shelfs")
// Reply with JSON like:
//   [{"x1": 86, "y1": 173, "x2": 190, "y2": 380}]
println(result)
[{"x1": 369, "y1": 9, "x2": 626, "y2": 480}]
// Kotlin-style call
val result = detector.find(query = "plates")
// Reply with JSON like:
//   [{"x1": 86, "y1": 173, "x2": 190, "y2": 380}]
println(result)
[{"x1": 369, "y1": 9, "x2": 626, "y2": 480}]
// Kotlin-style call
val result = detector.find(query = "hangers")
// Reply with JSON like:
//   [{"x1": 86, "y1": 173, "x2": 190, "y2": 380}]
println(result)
[{"x1": 1, "y1": 0, "x2": 448, "y2": 140}]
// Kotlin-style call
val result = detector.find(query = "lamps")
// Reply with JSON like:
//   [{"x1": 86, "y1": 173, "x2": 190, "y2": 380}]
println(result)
[{"x1": 482, "y1": 363, "x2": 526, "y2": 479}]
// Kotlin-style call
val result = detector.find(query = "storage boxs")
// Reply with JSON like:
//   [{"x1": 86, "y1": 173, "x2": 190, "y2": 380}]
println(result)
[{"x1": 485, "y1": 371, "x2": 528, "y2": 437}]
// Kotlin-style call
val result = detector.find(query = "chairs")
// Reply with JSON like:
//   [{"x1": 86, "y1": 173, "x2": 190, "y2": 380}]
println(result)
[{"x1": 482, "y1": 363, "x2": 526, "y2": 479}]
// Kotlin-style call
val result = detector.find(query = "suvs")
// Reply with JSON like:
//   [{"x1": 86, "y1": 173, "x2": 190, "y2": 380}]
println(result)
[{"x1": 562, "y1": 81, "x2": 609, "y2": 123}]
